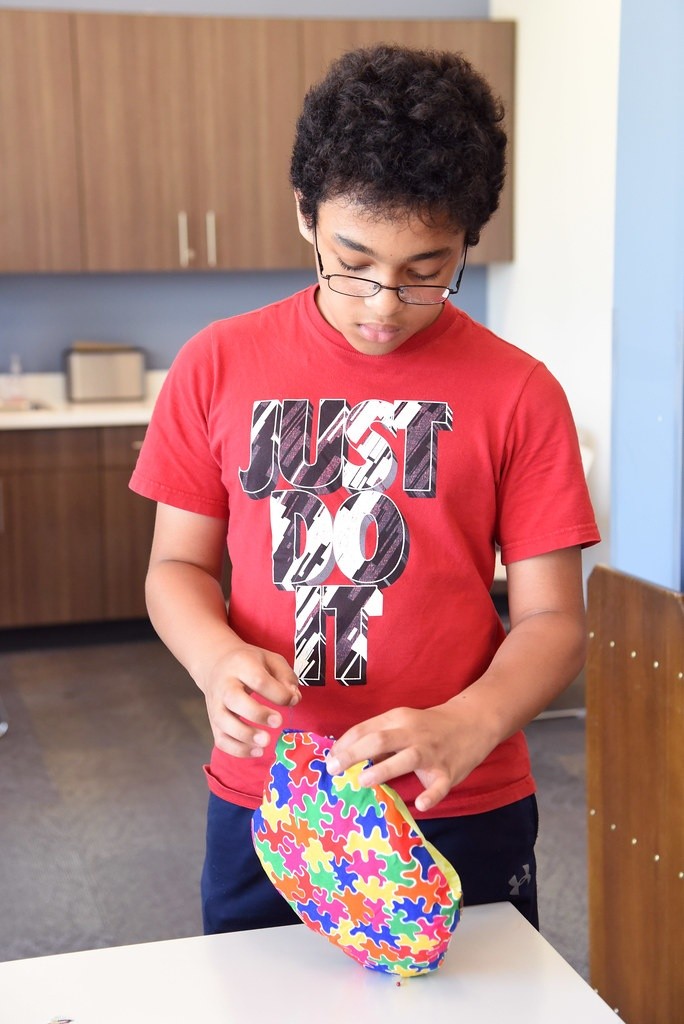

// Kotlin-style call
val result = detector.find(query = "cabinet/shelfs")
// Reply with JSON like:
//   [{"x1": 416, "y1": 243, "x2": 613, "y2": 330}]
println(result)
[
  {"x1": 0, "y1": 0, "x2": 516, "y2": 273},
  {"x1": 0, "y1": 428, "x2": 168, "y2": 642}
]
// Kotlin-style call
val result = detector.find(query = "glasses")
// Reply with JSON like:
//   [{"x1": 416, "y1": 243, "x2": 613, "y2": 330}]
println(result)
[{"x1": 312, "y1": 212, "x2": 468, "y2": 306}]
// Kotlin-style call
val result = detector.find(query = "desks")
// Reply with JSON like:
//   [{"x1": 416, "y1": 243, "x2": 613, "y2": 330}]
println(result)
[{"x1": 0, "y1": 901, "x2": 626, "y2": 1024}]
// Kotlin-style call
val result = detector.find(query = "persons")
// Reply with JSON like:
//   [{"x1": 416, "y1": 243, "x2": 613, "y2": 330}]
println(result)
[{"x1": 128, "y1": 46, "x2": 602, "y2": 935}]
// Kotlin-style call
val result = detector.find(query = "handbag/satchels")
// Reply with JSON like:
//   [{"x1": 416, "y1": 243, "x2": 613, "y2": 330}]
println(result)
[{"x1": 250, "y1": 729, "x2": 464, "y2": 979}]
